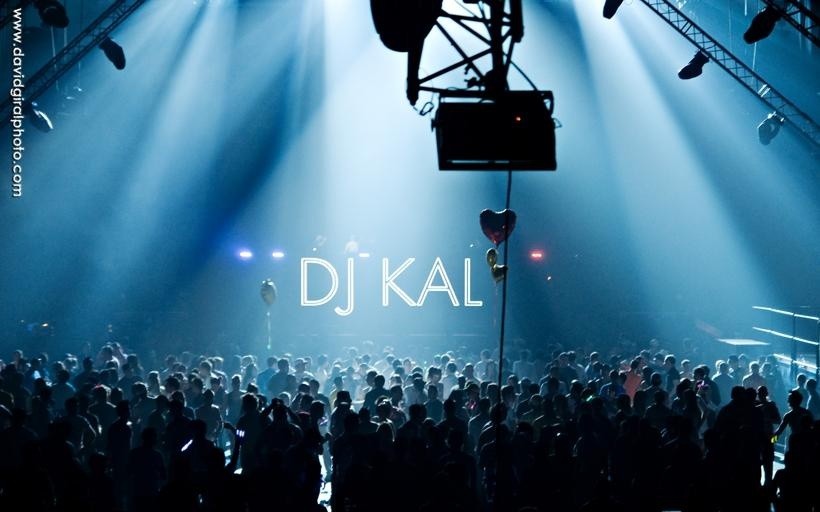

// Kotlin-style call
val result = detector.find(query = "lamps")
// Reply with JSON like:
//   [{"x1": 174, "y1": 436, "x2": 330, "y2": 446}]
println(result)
[
  {"x1": 17, "y1": 0, "x2": 128, "y2": 133},
  {"x1": 603, "y1": 0, "x2": 788, "y2": 147}
]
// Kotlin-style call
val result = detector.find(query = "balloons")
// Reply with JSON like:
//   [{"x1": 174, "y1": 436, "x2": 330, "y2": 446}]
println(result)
[
  {"x1": 479, "y1": 210, "x2": 517, "y2": 287},
  {"x1": 260, "y1": 278, "x2": 279, "y2": 307}
]
